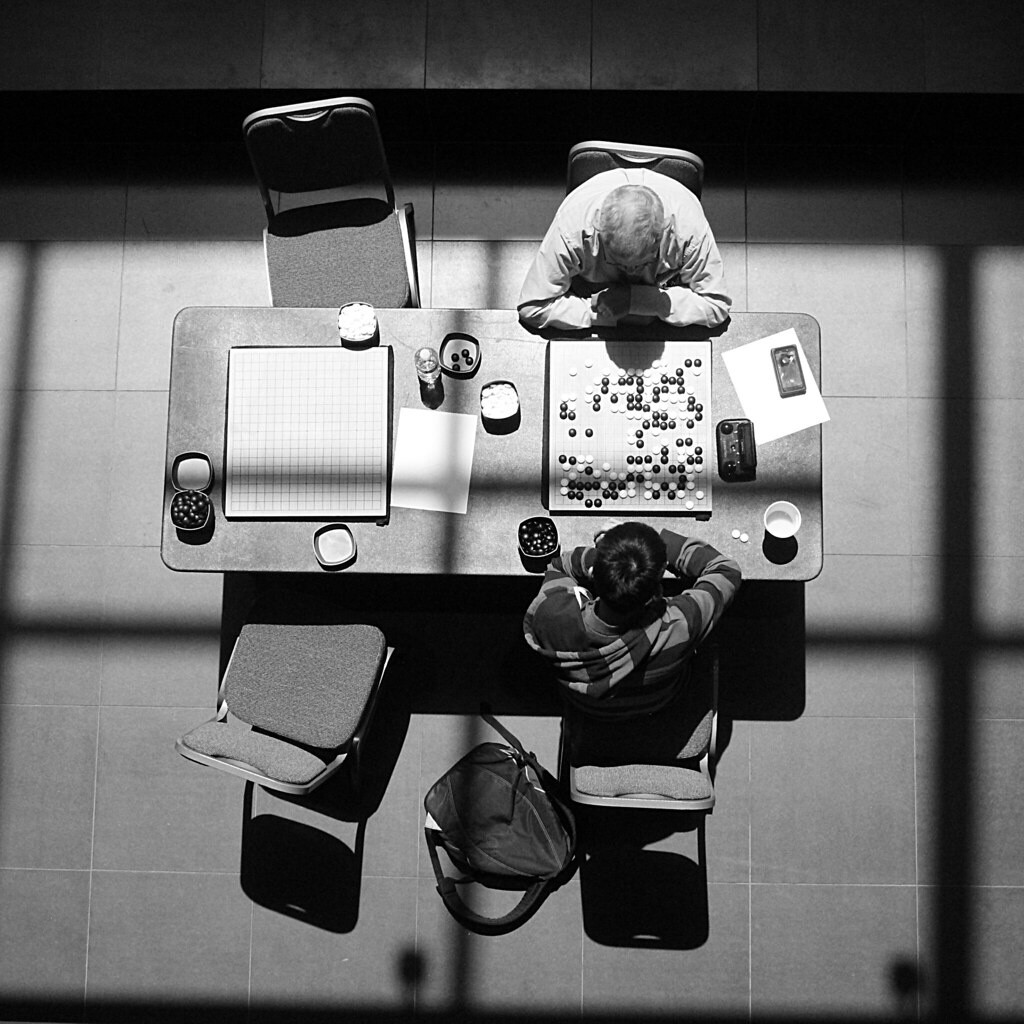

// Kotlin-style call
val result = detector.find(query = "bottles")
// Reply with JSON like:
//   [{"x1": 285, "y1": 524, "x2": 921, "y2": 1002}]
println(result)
[{"x1": 414, "y1": 347, "x2": 441, "y2": 383}]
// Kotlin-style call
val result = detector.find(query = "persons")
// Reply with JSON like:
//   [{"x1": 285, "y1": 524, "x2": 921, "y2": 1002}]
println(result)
[
  {"x1": 524, "y1": 522, "x2": 742, "y2": 720},
  {"x1": 516, "y1": 167, "x2": 731, "y2": 333}
]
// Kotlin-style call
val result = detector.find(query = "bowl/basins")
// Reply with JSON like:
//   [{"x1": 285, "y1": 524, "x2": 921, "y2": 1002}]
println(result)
[
  {"x1": 169, "y1": 451, "x2": 213, "y2": 530},
  {"x1": 516, "y1": 515, "x2": 559, "y2": 558},
  {"x1": 337, "y1": 302, "x2": 377, "y2": 341},
  {"x1": 312, "y1": 522, "x2": 357, "y2": 566},
  {"x1": 438, "y1": 332, "x2": 481, "y2": 373},
  {"x1": 480, "y1": 379, "x2": 520, "y2": 421}
]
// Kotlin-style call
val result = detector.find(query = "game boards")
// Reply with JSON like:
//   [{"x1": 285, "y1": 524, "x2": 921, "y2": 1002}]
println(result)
[
  {"x1": 223, "y1": 346, "x2": 388, "y2": 520},
  {"x1": 547, "y1": 338, "x2": 713, "y2": 513}
]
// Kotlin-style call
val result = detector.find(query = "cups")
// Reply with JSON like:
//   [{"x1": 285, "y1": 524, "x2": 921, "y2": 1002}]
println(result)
[{"x1": 763, "y1": 501, "x2": 801, "y2": 541}]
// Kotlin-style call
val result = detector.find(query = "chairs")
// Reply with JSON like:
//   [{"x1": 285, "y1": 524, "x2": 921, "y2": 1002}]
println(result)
[
  {"x1": 174, "y1": 604, "x2": 395, "y2": 795},
  {"x1": 567, "y1": 140, "x2": 705, "y2": 205},
  {"x1": 242, "y1": 96, "x2": 420, "y2": 310},
  {"x1": 557, "y1": 663, "x2": 719, "y2": 814}
]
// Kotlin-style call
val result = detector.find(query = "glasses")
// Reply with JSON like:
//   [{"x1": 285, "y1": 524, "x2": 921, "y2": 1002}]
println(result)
[{"x1": 599, "y1": 234, "x2": 660, "y2": 270}]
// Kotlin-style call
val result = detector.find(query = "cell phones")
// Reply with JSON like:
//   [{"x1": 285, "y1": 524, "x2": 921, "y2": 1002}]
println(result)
[{"x1": 771, "y1": 344, "x2": 806, "y2": 397}]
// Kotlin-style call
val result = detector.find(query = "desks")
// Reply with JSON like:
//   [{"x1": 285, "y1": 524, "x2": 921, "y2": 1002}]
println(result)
[{"x1": 159, "y1": 306, "x2": 826, "y2": 582}]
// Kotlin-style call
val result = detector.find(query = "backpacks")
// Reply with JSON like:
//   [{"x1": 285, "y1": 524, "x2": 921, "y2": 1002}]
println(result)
[{"x1": 424, "y1": 698, "x2": 578, "y2": 925}]
